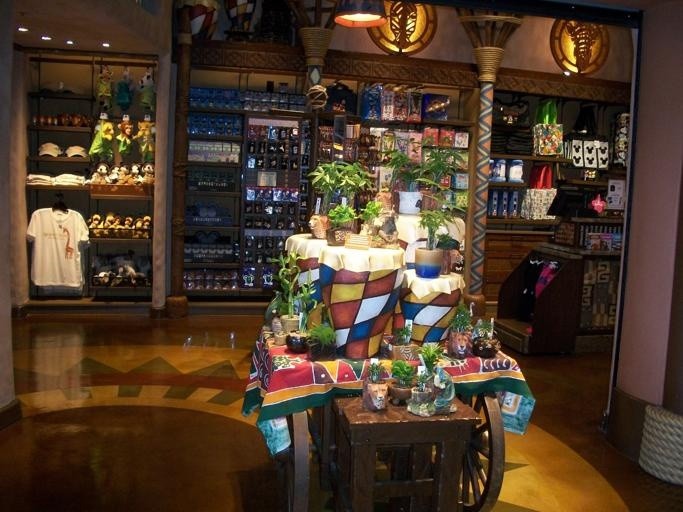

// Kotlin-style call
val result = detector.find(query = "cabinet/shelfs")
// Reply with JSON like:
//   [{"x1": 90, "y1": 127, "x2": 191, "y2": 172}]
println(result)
[
  {"x1": 482, "y1": 89, "x2": 626, "y2": 336},
  {"x1": 25, "y1": 46, "x2": 159, "y2": 299},
  {"x1": 317, "y1": 49, "x2": 476, "y2": 214},
  {"x1": 181, "y1": 39, "x2": 317, "y2": 298}
]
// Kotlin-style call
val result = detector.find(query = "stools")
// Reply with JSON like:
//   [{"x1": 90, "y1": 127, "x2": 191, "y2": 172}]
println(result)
[{"x1": 332, "y1": 386, "x2": 482, "y2": 512}]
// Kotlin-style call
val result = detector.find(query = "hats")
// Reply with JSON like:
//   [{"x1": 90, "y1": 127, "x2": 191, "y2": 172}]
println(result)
[
  {"x1": 38, "y1": 143, "x2": 63, "y2": 158},
  {"x1": 65, "y1": 145, "x2": 88, "y2": 158}
]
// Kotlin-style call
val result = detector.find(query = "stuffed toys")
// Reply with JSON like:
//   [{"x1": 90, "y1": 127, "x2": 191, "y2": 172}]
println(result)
[{"x1": 88, "y1": 63, "x2": 156, "y2": 288}]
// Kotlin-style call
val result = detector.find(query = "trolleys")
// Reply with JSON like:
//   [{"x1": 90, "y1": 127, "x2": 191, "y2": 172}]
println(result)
[{"x1": 291, "y1": 390, "x2": 504, "y2": 512}]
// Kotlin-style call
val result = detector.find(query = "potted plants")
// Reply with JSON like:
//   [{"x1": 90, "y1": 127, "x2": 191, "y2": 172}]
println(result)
[
  {"x1": 363, "y1": 305, "x2": 498, "y2": 412},
  {"x1": 264, "y1": 251, "x2": 336, "y2": 361},
  {"x1": 416, "y1": 210, "x2": 455, "y2": 278},
  {"x1": 307, "y1": 135, "x2": 412, "y2": 246},
  {"x1": 435, "y1": 234, "x2": 451, "y2": 274},
  {"x1": 412, "y1": 135, "x2": 469, "y2": 215}
]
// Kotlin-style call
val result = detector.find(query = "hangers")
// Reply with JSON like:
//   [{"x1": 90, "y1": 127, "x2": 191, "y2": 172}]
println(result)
[{"x1": 50, "y1": 194, "x2": 69, "y2": 213}]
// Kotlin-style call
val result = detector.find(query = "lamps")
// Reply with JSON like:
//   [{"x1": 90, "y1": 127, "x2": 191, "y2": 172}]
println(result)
[{"x1": 333, "y1": 0, "x2": 387, "y2": 29}]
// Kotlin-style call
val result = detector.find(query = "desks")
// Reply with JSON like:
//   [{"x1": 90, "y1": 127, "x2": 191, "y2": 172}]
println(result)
[{"x1": 242, "y1": 319, "x2": 536, "y2": 512}]
